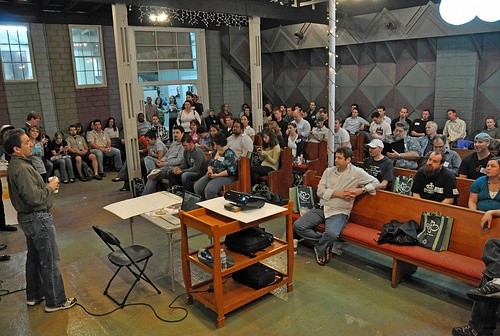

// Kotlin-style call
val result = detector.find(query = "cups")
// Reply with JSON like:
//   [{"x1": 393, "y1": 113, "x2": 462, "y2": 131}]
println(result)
[
  {"x1": 293, "y1": 239, "x2": 298, "y2": 254},
  {"x1": 48, "y1": 176, "x2": 59, "y2": 193}
]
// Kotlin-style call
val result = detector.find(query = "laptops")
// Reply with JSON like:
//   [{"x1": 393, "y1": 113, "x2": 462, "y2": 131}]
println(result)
[{"x1": 161, "y1": 190, "x2": 202, "y2": 225}]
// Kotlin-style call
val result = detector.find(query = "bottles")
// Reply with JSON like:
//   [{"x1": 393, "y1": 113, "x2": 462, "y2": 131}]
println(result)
[{"x1": 220, "y1": 249, "x2": 227, "y2": 272}]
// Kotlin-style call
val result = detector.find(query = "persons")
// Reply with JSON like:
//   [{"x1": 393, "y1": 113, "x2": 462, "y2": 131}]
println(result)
[
  {"x1": 194, "y1": 133, "x2": 239, "y2": 201},
  {"x1": 0, "y1": 179, "x2": 17, "y2": 262},
  {"x1": 144, "y1": 90, "x2": 204, "y2": 135},
  {"x1": 452, "y1": 208, "x2": 500, "y2": 336},
  {"x1": 411, "y1": 150, "x2": 460, "y2": 206},
  {"x1": 3, "y1": 128, "x2": 77, "y2": 313},
  {"x1": 293, "y1": 146, "x2": 381, "y2": 265},
  {"x1": 141, "y1": 126, "x2": 186, "y2": 197},
  {"x1": 361, "y1": 138, "x2": 395, "y2": 193},
  {"x1": 380, "y1": 108, "x2": 500, "y2": 180},
  {"x1": 0, "y1": 111, "x2": 127, "y2": 184},
  {"x1": 251, "y1": 103, "x2": 329, "y2": 193},
  {"x1": 188, "y1": 102, "x2": 255, "y2": 160},
  {"x1": 468, "y1": 157, "x2": 500, "y2": 212},
  {"x1": 110, "y1": 112, "x2": 168, "y2": 190},
  {"x1": 166, "y1": 134, "x2": 209, "y2": 193},
  {"x1": 334, "y1": 104, "x2": 392, "y2": 158}
]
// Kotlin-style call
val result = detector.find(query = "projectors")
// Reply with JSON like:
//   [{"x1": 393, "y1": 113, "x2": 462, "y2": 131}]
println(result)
[{"x1": 223, "y1": 190, "x2": 266, "y2": 209}]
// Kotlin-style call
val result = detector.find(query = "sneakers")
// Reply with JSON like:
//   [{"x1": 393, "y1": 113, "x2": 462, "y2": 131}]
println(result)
[
  {"x1": 27, "y1": 296, "x2": 46, "y2": 306},
  {"x1": 451, "y1": 325, "x2": 482, "y2": 336},
  {"x1": 45, "y1": 296, "x2": 78, "y2": 311},
  {"x1": 465, "y1": 279, "x2": 500, "y2": 301}
]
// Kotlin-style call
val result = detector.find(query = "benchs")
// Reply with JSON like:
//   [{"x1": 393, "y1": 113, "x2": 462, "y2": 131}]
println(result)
[
  {"x1": 146, "y1": 144, "x2": 251, "y2": 201},
  {"x1": 266, "y1": 128, "x2": 500, "y2": 290}
]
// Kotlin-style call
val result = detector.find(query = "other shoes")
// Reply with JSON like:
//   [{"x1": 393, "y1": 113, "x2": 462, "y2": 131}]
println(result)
[
  {"x1": 147, "y1": 169, "x2": 162, "y2": 180},
  {"x1": 119, "y1": 187, "x2": 130, "y2": 191},
  {"x1": 0, "y1": 243, "x2": 7, "y2": 249},
  {"x1": 112, "y1": 178, "x2": 124, "y2": 182},
  {"x1": 63, "y1": 172, "x2": 105, "y2": 184},
  {"x1": 324, "y1": 244, "x2": 333, "y2": 262},
  {"x1": 315, "y1": 246, "x2": 325, "y2": 266},
  {"x1": 0, "y1": 255, "x2": 9, "y2": 261},
  {"x1": 0, "y1": 225, "x2": 18, "y2": 232}
]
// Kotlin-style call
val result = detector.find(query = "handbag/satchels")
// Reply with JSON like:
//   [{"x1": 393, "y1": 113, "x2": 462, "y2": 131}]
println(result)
[
  {"x1": 374, "y1": 210, "x2": 454, "y2": 252},
  {"x1": 224, "y1": 226, "x2": 273, "y2": 257},
  {"x1": 392, "y1": 175, "x2": 413, "y2": 197},
  {"x1": 232, "y1": 262, "x2": 284, "y2": 290},
  {"x1": 289, "y1": 185, "x2": 314, "y2": 213},
  {"x1": 250, "y1": 151, "x2": 260, "y2": 166},
  {"x1": 184, "y1": 190, "x2": 201, "y2": 211}
]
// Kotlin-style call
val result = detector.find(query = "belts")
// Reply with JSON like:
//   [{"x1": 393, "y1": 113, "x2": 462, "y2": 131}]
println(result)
[{"x1": 18, "y1": 209, "x2": 50, "y2": 215}]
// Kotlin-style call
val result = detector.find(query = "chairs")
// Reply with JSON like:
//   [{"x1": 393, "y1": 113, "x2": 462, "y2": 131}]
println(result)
[{"x1": 89, "y1": 224, "x2": 162, "y2": 309}]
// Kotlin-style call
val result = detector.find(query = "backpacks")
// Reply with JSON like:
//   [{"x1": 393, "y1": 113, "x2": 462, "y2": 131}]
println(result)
[{"x1": 82, "y1": 161, "x2": 93, "y2": 180}]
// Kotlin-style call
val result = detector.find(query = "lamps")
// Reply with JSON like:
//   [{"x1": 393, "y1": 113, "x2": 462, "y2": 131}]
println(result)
[
  {"x1": 19, "y1": 65, "x2": 25, "y2": 69},
  {"x1": 86, "y1": 59, "x2": 97, "y2": 63},
  {"x1": 72, "y1": 42, "x2": 88, "y2": 49}
]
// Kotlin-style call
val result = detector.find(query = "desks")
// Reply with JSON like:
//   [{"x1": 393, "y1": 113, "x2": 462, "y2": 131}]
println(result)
[
  {"x1": 126, "y1": 191, "x2": 214, "y2": 294},
  {"x1": 177, "y1": 199, "x2": 294, "y2": 330}
]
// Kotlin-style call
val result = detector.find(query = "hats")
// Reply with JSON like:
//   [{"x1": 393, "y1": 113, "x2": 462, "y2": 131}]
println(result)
[
  {"x1": 474, "y1": 133, "x2": 491, "y2": 141},
  {"x1": 366, "y1": 139, "x2": 384, "y2": 148}
]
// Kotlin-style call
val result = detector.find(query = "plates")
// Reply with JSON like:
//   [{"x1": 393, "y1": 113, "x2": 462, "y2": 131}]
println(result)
[{"x1": 152, "y1": 209, "x2": 168, "y2": 216}]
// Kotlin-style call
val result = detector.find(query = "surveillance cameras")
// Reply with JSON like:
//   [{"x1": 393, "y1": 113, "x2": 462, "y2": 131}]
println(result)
[{"x1": 388, "y1": 22, "x2": 395, "y2": 28}]
